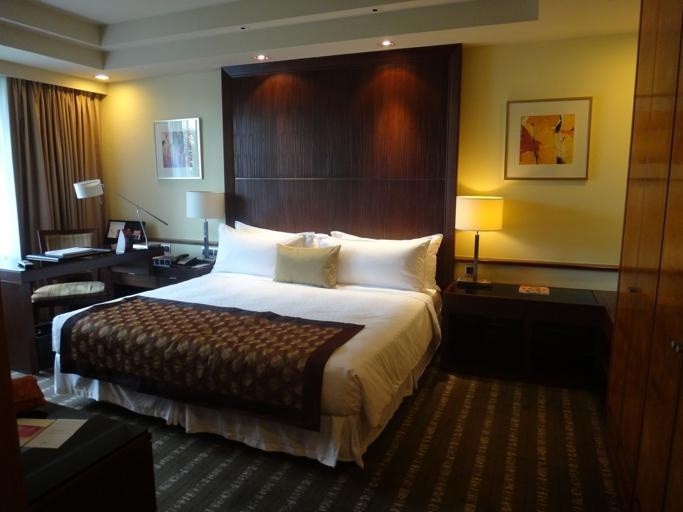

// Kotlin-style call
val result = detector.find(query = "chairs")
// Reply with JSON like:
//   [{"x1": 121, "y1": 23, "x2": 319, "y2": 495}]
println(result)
[{"x1": 31, "y1": 228, "x2": 107, "y2": 326}]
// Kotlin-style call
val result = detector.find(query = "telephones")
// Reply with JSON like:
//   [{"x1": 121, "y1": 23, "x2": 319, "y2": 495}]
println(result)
[{"x1": 173, "y1": 253, "x2": 197, "y2": 265}]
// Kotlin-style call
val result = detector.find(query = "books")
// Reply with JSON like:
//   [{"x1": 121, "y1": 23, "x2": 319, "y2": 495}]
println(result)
[
  {"x1": 517, "y1": 284, "x2": 551, "y2": 297},
  {"x1": 15, "y1": 415, "x2": 89, "y2": 453}
]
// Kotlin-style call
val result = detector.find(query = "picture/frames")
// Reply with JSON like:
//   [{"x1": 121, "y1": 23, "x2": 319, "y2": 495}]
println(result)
[
  {"x1": 153, "y1": 118, "x2": 202, "y2": 180},
  {"x1": 504, "y1": 96, "x2": 593, "y2": 180}
]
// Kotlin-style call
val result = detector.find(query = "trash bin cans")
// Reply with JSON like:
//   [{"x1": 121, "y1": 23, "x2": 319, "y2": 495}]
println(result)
[{"x1": 33, "y1": 321, "x2": 56, "y2": 370}]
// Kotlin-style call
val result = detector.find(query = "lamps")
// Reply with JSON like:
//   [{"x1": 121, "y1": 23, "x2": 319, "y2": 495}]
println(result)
[
  {"x1": 454, "y1": 195, "x2": 503, "y2": 289},
  {"x1": 74, "y1": 179, "x2": 168, "y2": 251},
  {"x1": 187, "y1": 191, "x2": 225, "y2": 261}
]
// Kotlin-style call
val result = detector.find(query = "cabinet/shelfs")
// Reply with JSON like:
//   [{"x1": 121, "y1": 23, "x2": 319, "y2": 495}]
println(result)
[{"x1": 604, "y1": 0, "x2": 683, "y2": 512}]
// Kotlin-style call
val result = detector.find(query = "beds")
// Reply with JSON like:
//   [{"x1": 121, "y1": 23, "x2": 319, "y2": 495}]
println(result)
[{"x1": 52, "y1": 178, "x2": 445, "y2": 469}]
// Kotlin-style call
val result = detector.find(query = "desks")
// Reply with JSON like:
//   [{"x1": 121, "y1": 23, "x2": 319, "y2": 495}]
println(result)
[
  {"x1": 0, "y1": 245, "x2": 164, "y2": 375},
  {"x1": 0, "y1": 399, "x2": 158, "y2": 512}
]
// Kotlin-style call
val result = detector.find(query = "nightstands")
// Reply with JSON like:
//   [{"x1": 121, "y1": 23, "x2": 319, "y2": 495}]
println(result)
[
  {"x1": 439, "y1": 281, "x2": 606, "y2": 390},
  {"x1": 110, "y1": 259, "x2": 215, "y2": 300}
]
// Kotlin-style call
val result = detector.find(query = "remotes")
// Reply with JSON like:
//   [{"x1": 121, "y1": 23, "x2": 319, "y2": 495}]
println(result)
[
  {"x1": 18, "y1": 260, "x2": 34, "y2": 268},
  {"x1": 178, "y1": 255, "x2": 197, "y2": 265}
]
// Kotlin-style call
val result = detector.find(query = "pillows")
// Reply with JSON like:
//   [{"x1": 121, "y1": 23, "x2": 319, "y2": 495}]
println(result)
[{"x1": 210, "y1": 221, "x2": 443, "y2": 291}]
